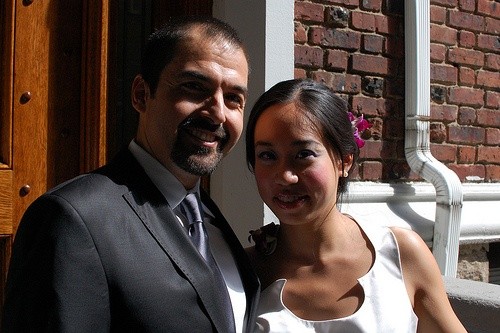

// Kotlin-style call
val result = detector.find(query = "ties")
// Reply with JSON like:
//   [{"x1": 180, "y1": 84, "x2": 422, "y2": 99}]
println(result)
[{"x1": 178, "y1": 192, "x2": 236, "y2": 333}]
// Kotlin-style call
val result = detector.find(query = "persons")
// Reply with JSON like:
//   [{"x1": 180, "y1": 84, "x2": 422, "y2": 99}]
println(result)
[
  {"x1": 1, "y1": 14, "x2": 262, "y2": 333},
  {"x1": 243, "y1": 78, "x2": 470, "y2": 333}
]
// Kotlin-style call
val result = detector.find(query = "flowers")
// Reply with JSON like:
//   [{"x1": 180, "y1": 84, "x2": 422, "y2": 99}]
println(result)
[{"x1": 348, "y1": 112, "x2": 373, "y2": 148}]
